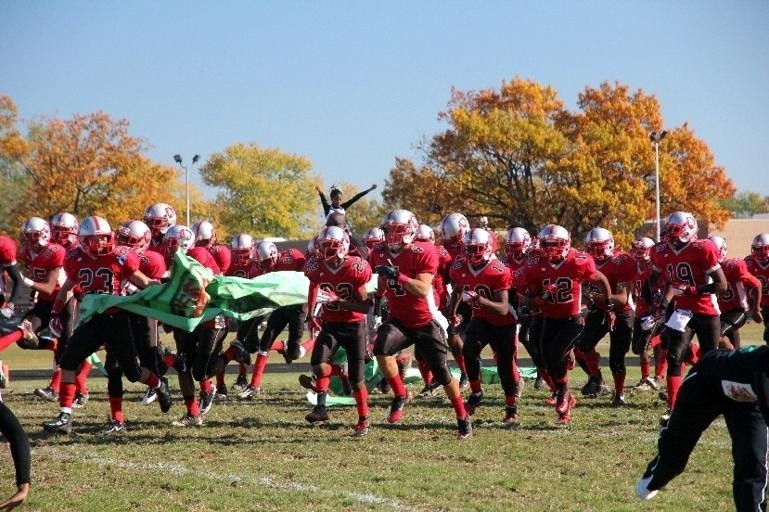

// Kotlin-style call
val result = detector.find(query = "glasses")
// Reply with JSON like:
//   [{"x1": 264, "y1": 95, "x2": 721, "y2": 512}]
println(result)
[{"x1": 326, "y1": 221, "x2": 347, "y2": 226}]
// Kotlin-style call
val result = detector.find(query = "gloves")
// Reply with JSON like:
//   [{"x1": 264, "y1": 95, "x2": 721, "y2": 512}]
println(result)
[
  {"x1": 522, "y1": 296, "x2": 544, "y2": 315},
  {"x1": 303, "y1": 316, "x2": 320, "y2": 339},
  {"x1": 0, "y1": 301, "x2": 15, "y2": 321},
  {"x1": 603, "y1": 309, "x2": 616, "y2": 331},
  {"x1": 641, "y1": 315, "x2": 657, "y2": 331},
  {"x1": 449, "y1": 316, "x2": 462, "y2": 327},
  {"x1": 752, "y1": 303, "x2": 764, "y2": 324},
  {"x1": 375, "y1": 258, "x2": 400, "y2": 281},
  {"x1": 672, "y1": 279, "x2": 690, "y2": 297},
  {"x1": 316, "y1": 288, "x2": 338, "y2": 305},
  {"x1": 652, "y1": 292, "x2": 664, "y2": 307},
  {"x1": 462, "y1": 290, "x2": 479, "y2": 302},
  {"x1": 49, "y1": 313, "x2": 65, "y2": 338}
]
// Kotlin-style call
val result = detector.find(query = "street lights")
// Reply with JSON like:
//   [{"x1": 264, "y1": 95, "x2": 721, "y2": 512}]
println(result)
[
  {"x1": 649, "y1": 128, "x2": 667, "y2": 244},
  {"x1": 174, "y1": 152, "x2": 200, "y2": 230}
]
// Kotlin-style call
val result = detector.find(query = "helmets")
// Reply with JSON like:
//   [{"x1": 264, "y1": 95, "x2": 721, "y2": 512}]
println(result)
[
  {"x1": 418, "y1": 224, "x2": 436, "y2": 245},
  {"x1": 254, "y1": 241, "x2": 278, "y2": 273},
  {"x1": 316, "y1": 226, "x2": 350, "y2": 262},
  {"x1": 504, "y1": 227, "x2": 530, "y2": 259},
  {"x1": 709, "y1": 235, "x2": 728, "y2": 263},
  {"x1": 304, "y1": 239, "x2": 315, "y2": 259},
  {"x1": 143, "y1": 203, "x2": 177, "y2": 237},
  {"x1": 192, "y1": 221, "x2": 217, "y2": 250},
  {"x1": 525, "y1": 241, "x2": 540, "y2": 260},
  {"x1": 231, "y1": 234, "x2": 255, "y2": 267},
  {"x1": 24, "y1": 217, "x2": 51, "y2": 251},
  {"x1": 48, "y1": 212, "x2": 79, "y2": 243},
  {"x1": 751, "y1": 233, "x2": 769, "y2": 263},
  {"x1": 460, "y1": 228, "x2": 493, "y2": 265},
  {"x1": 663, "y1": 210, "x2": 697, "y2": 243},
  {"x1": 162, "y1": 224, "x2": 195, "y2": 256},
  {"x1": 632, "y1": 237, "x2": 655, "y2": 260},
  {"x1": 362, "y1": 226, "x2": 385, "y2": 248},
  {"x1": 539, "y1": 224, "x2": 572, "y2": 261},
  {"x1": 440, "y1": 213, "x2": 471, "y2": 253},
  {"x1": 379, "y1": 209, "x2": 419, "y2": 249},
  {"x1": 76, "y1": 216, "x2": 115, "y2": 257},
  {"x1": 584, "y1": 226, "x2": 614, "y2": 261},
  {"x1": 114, "y1": 221, "x2": 151, "y2": 250}
]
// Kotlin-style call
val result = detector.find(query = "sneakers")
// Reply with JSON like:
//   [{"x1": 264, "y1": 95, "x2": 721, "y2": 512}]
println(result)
[
  {"x1": 237, "y1": 384, "x2": 260, "y2": 399},
  {"x1": 430, "y1": 377, "x2": 441, "y2": 388},
  {"x1": 396, "y1": 352, "x2": 413, "y2": 380},
  {"x1": 636, "y1": 378, "x2": 651, "y2": 389},
  {"x1": 504, "y1": 405, "x2": 517, "y2": 423},
  {"x1": 459, "y1": 373, "x2": 469, "y2": 389},
  {"x1": 164, "y1": 347, "x2": 188, "y2": 373},
  {"x1": 231, "y1": 374, "x2": 249, "y2": 390},
  {"x1": 659, "y1": 391, "x2": 668, "y2": 401},
  {"x1": 43, "y1": 412, "x2": 71, "y2": 434},
  {"x1": 99, "y1": 416, "x2": 126, "y2": 433},
  {"x1": 278, "y1": 340, "x2": 292, "y2": 365},
  {"x1": 169, "y1": 413, "x2": 203, "y2": 427},
  {"x1": 34, "y1": 386, "x2": 58, "y2": 402},
  {"x1": 612, "y1": 393, "x2": 626, "y2": 405},
  {"x1": 566, "y1": 349, "x2": 575, "y2": 370},
  {"x1": 659, "y1": 409, "x2": 673, "y2": 427},
  {"x1": 387, "y1": 390, "x2": 414, "y2": 423},
  {"x1": 457, "y1": 414, "x2": 472, "y2": 439},
  {"x1": 0, "y1": 359, "x2": 8, "y2": 388},
  {"x1": 581, "y1": 371, "x2": 602, "y2": 395},
  {"x1": 142, "y1": 387, "x2": 158, "y2": 405},
  {"x1": 468, "y1": 389, "x2": 484, "y2": 416},
  {"x1": 299, "y1": 375, "x2": 318, "y2": 393},
  {"x1": 545, "y1": 391, "x2": 559, "y2": 407},
  {"x1": 17, "y1": 318, "x2": 40, "y2": 346},
  {"x1": 646, "y1": 376, "x2": 662, "y2": 391},
  {"x1": 154, "y1": 377, "x2": 172, "y2": 412},
  {"x1": 72, "y1": 390, "x2": 89, "y2": 408},
  {"x1": 589, "y1": 381, "x2": 611, "y2": 398},
  {"x1": 381, "y1": 377, "x2": 392, "y2": 393},
  {"x1": 355, "y1": 415, "x2": 370, "y2": 433},
  {"x1": 305, "y1": 404, "x2": 328, "y2": 423},
  {"x1": 637, "y1": 474, "x2": 659, "y2": 501},
  {"x1": 198, "y1": 384, "x2": 217, "y2": 415},
  {"x1": 338, "y1": 362, "x2": 352, "y2": 396},
  {"x1": 216, "y1": 384, "x2": 227, "y2": 399},
  {"x1": 230, "y1": 337, "x2": 250, "y2": 365},
  {"x1": 534, "y1": 375, "x2": 546, "y2": 389},
  {"x1": 559, "y1": 394, "x2": 576, "y2": 422},
  {"x1": 556, "y1": 389, "x2": 569, "y2": 413},
  {"x1": 418, "y1": 381, "x2": 442, "y2": 399}
]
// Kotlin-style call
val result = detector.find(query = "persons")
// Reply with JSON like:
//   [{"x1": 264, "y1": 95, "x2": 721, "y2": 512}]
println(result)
[
  {"x1": 637, "y1": 342, "x2": 767, "y2": 512},
  {"x1": 0, "y1": 403, "x2": 31, "y2": 512},
  {"x1": 2, "y1": 184, "x2": 769, "y2": 437}
]
